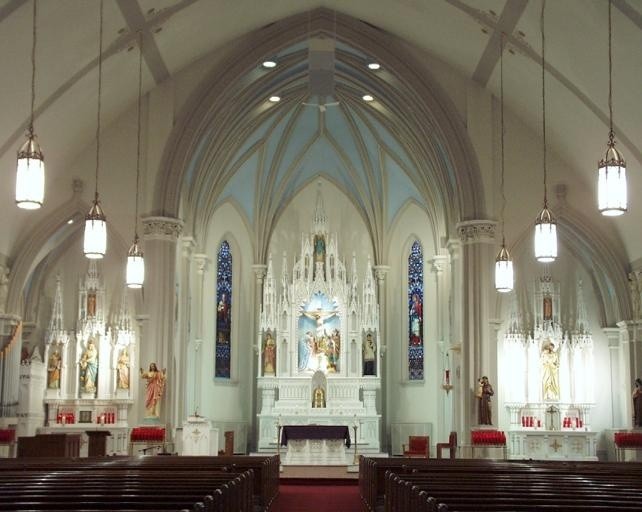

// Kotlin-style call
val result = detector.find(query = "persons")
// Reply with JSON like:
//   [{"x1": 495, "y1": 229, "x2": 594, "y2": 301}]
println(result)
[
  {"x1": 218, "y1": 293, "x2": 229, "y2": 324},
  {"x1": 262, "y1": 332, "x2": 276, "y2": 375},
  {"x1": 362, "y1": 333, "x2": 376, "y2": 374},
  {"x1": 478, "y1": 375, "x2": 494, "y2": 425},
  {"x1": 409, "y1": 294, "x2": 422, "y2": 346},
  {"x1": 80, "y1": 338, "x2": 98, "y2": 393},
  {"x1": 140, "y1": 362, "x2": 166, "y2": 416},
  {"x1": 117, "y1": 347, "x2": 130, "y2": 389},
  {"x1": 48, "y1": 350, "x2": 61, "y2": 387},
  {"x1": 631, "y1": 378, "x2": 642, "y2": 427}
]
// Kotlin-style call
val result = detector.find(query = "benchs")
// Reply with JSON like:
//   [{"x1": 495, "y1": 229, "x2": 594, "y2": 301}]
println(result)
[
  {"x1": 0, "y1": 425, "x2": 280, "y2": 512},
  {"x1": 356, "y1": 429, "x2": 642, "y2": 512}
]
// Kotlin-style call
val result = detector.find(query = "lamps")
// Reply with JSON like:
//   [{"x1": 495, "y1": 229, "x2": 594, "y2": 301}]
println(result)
[
  {"x1": 76, "y1": 0, "x2": 113, "y2": 260},
  {"x1": 489, "y1": 26, "x2": 518, "y2": 295},
  {"x1": 14, "y1": 0, "x2": 48, "y2": 211},
  {"x1": 526, "y1": 0, "x2": 559, "y2": 267},
  {"x1": 593, "y1": 1, "x2": 630, "y2": 219},
  {"x1": 122, "y1": 27, "x2": 146, "y2": 291}
]
